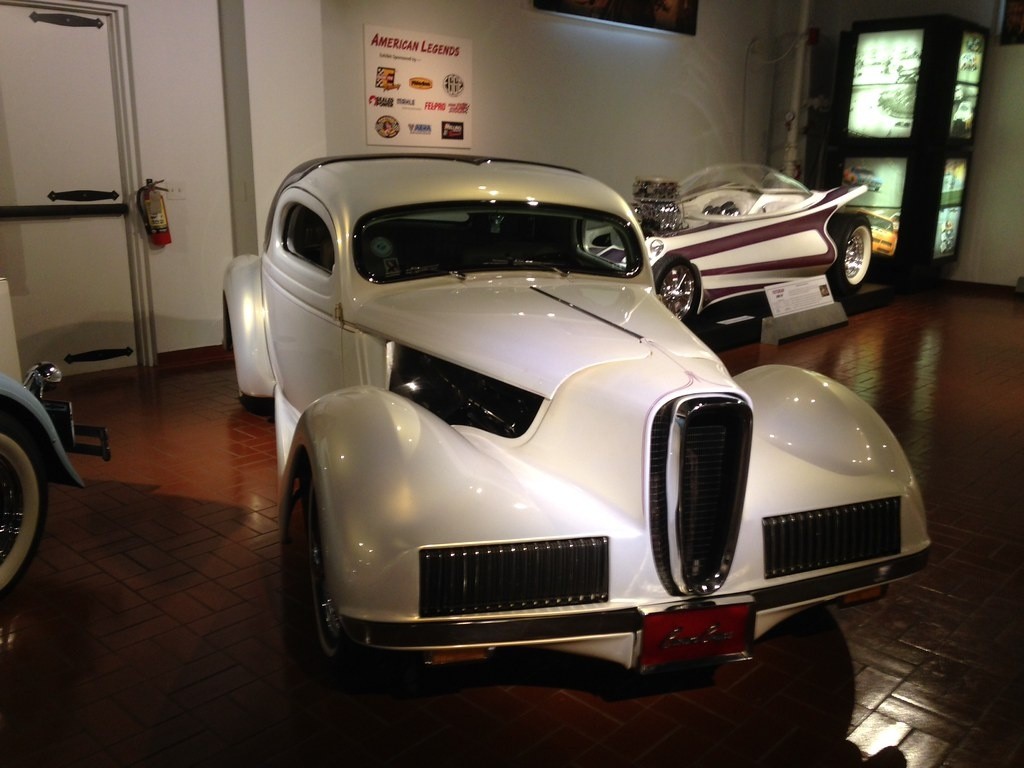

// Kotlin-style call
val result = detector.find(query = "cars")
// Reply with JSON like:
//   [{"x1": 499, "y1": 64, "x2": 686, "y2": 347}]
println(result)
[
  {"x1": 844, "y1": 166, "x2": 883, "y2": 192},
  {"x1": 853, "y1": 206, "x2": 898, "y2": 256},
  {"x1": 582, "y1": 160, "x2": 874, "y2": 319},
  {"x1": 0, "y1": 363, "x2": 112, "y2": 594},
  {"x1": 961, "y1": 53, "x2": 977, "y2": 69},
  {"x1": 223, "y1": 152, "x2": 934, "y2": 676},
  {"x1": 967, "y1": 37, "x2": 983, "y2": 53},
  {"x1": 954, "y1": 102, "x2": 974, "y2": 128}
]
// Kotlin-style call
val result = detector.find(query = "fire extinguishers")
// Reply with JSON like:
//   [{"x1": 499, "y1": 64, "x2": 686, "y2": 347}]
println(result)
[{"x1": 137, "y1": 178, "x2": 172, "y2": 245}]
